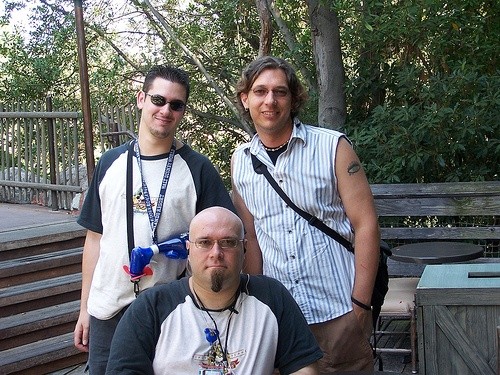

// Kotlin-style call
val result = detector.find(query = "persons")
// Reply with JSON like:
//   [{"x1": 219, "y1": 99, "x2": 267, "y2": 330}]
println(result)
[
  {"x1": 232, "y1": 56, "x2": 393, "y2": 375},
  {"x1": 104, "y1": 206, "x2": 323, "y2": 375},
  {"x1": 73, "y1": 64, "x2": 247, "y2": 375}
]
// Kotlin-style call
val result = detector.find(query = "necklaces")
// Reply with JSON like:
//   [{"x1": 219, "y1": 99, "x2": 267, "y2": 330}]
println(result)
[
  {"x1": 259, "y1": 139, "x2": 290, "y2": 151},
  {"x1": 191, "y1": 292, "x2": 240, "y2": 366}
]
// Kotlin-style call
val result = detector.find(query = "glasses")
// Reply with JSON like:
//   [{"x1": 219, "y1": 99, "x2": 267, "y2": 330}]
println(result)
[
  {"x1": 187, "y1": 237, "x2": 245, "y2": 249},
  {"x1": 143, "y1": 91, "x2": 186, "y2": 111}
]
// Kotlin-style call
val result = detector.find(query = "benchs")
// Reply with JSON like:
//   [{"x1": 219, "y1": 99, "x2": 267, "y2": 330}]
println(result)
[{"x1": 368, "y1": 181, "x2": 500, "y2": 276}]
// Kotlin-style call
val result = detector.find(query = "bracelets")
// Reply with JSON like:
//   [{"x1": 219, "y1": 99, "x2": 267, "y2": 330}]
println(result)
[{"x1": 349, "y1": 295, "x2": 376, "y2": 313}]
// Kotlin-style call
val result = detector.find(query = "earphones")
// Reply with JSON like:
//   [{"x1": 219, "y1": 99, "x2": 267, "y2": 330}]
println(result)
[
  {"x1": 244, "y1": 247, "x2": 246, "y2": 253},
  {"x1": 187, "y1": 249, "x2": 189, "y2": 255}
]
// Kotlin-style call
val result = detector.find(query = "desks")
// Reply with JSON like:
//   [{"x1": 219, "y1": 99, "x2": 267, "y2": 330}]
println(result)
[{"x1": 387, "y1": 242, "x2": 484, "y2": 263}]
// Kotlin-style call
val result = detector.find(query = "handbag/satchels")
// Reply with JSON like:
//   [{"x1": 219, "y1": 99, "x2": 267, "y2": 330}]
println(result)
[{"x1": 372, "y1": 239, "x2": 392, "y2": 323}]
